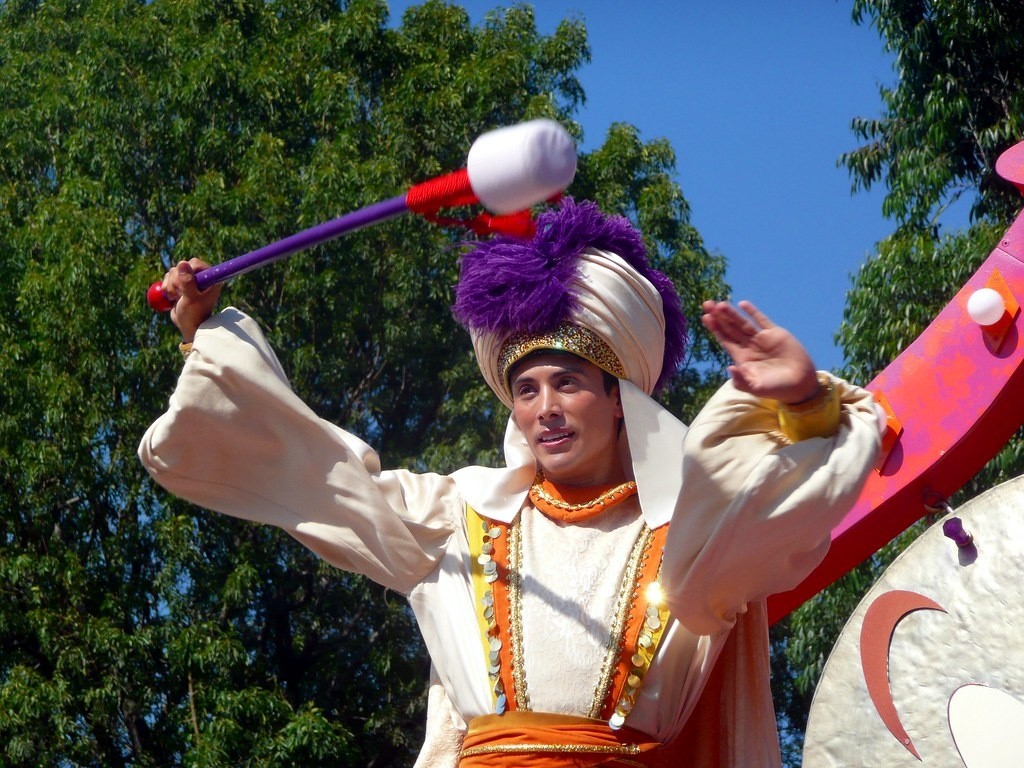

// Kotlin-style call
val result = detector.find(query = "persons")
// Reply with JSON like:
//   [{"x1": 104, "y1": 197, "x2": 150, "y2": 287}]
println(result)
[{"x1": 134, "y1": 257, "x2": 884, "y2": 768}]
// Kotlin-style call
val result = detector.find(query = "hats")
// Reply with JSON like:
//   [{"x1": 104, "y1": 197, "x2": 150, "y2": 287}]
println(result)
[{"x1": 450, "y1": 194, "x2": 689, "y2": 410}]
